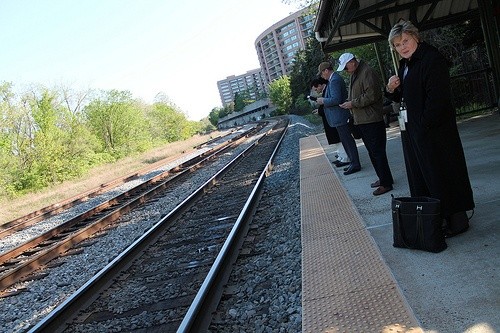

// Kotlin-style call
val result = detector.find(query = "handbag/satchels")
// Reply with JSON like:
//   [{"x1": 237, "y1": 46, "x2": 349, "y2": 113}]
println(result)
[
  {"x1": 346, "y1": 115, "x2": 364, "y2": 139},
  {"x1": 390, "y1": 194, "x2": 449, "y2": 254}
]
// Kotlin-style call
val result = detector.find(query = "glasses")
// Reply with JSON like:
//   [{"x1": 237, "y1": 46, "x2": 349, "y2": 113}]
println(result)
[
  {"x1": 394, "y1": 38, "x2": 413, "y2": 49},
  {"x1": 343, "y1": 68, "x2": 348, "y2": 71}
]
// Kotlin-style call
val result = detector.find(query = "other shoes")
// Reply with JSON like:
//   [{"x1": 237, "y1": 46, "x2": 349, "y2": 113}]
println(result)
[
  {"x1": 335, "y1": 154, "x2": 339, "y2": 158},
  {"x1": 335, "y1": 161, "x2": 350, "y2": 167},
  {"x1": 443, "y1": 219, "x2": 469, "y2": 240},
  {"x1": 332, "y1": 160, "x2": 340, "y2": 164}
]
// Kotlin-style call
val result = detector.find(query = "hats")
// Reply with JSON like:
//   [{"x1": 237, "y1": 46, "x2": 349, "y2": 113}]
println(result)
[
  {"x1": 336, "y1": 52, "x2": 354, "y2": 72},
  {"x1": 315, "y1": 62, "x2": 333, "y2": 77}
]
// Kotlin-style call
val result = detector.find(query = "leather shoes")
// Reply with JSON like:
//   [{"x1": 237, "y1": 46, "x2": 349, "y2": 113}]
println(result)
[
  {"x1": 373, "y1": 185, "x2": 393, "y2": 196},
  {"x1": 343, "y1": 166, "x2": 350, "y2": 171},
  {"x1": 343, "y1": 165, "x2": 359, "y2": 175},
  {"x1": 371, "y1": 180, "x2": 381, "y2": 188}
]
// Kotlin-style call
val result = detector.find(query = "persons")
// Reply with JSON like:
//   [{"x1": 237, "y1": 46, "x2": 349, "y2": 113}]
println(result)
[
  {"x1": 336, "y1": 53, "x2": 395, "y2": 196},
  {"x1": 384, "y1": 20, "x2": 475, "y2": 238},
  {"x1": 316, "y1": 62, "x2": 361, "y2": 175},
  {"x1": 311, "y1": 79, "x2": 351, "y2": 168}
]
